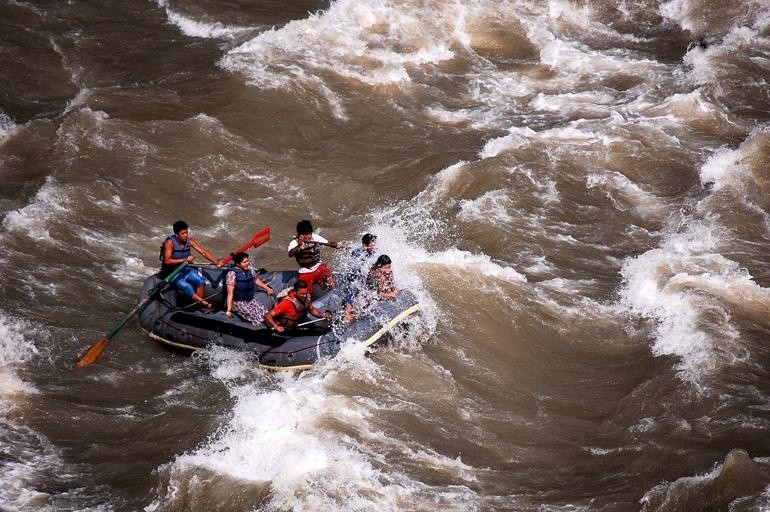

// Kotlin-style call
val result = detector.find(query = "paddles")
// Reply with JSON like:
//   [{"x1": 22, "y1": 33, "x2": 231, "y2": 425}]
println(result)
[
  {"x1": 244, "y1": 318, "x2": 327, "y2": 343},
  {"x1": 219, "y1": 227, "x2": 270, "y2": 268},
  {"x1": 77, "y1": 255, "x2": 196, "y2": 367}
]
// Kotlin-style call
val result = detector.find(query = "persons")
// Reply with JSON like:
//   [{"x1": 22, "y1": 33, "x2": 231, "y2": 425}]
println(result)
[
  {"x1": 159, "y1": 219, "x2": 223, "y2": 310},
  {"x1": 365, "y1": 254, "x2": 401, "y2": 300},
  {"x1": 348, "y1": 232, "x2": 378, "y2": 271},
  {"x1": 340, "y1": 286, "x2": 361, "y2": 324},
  {"x1": 287, "y1": 218, "x2": 345, "y2": 297},
  {"x1": 218, "y1": 252, "x2": 278, "y2": 331},
  {"x1": 262, "y1": 280, "x2": 333, "y2": 336}
]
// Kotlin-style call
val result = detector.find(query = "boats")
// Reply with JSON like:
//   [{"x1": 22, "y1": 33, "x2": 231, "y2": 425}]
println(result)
[{"x1": 138, "y1": 264, "x2": 422, "y2": 372}]
